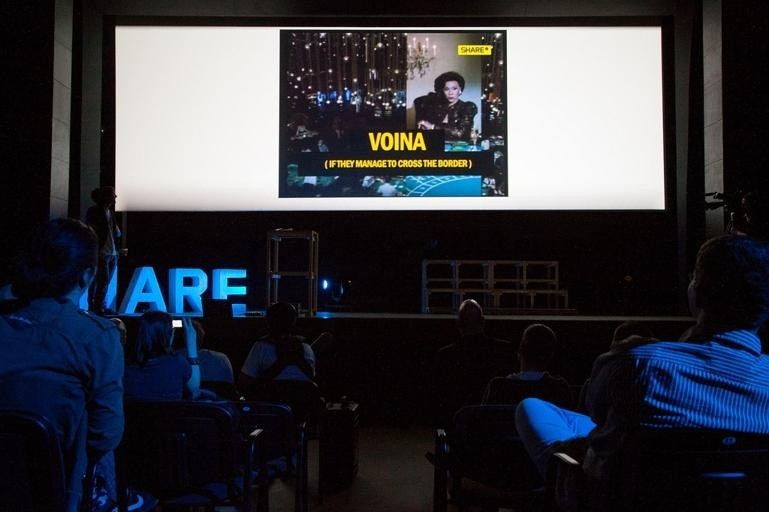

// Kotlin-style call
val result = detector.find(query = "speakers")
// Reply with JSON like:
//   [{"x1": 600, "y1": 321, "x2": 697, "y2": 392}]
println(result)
[{"x1": 316, "y1": 401, "x2": 360, "y2": 489}]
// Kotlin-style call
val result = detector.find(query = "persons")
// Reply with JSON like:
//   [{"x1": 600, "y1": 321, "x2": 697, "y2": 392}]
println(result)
[
  {"x1": 0, "y1": 183, "x2": 316, "y2": 512},
  {"x1": 415, "y1": 70, "x2": 479, "y2": 145},
  {"x1": 424, "y1": 233, "x2": 769, "y2": 512}
]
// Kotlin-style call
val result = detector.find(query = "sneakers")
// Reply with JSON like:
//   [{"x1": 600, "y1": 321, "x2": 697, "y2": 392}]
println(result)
[
  {"x1": 96, "y1": 486, "x2": 159, "y2": 511},
  {"x1": 425, "y1": 449, "x2": 459, "y2": 471}
]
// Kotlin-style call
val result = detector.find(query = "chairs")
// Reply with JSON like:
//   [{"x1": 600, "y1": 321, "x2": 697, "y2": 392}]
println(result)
[
  {"x1": 425, "y1": 363, "x2": 769, "y2": 509},
  {"x1": 0, "y1": 376, "x2": 326, "y2": 511},
  {"x1": 422, "y1": 260, "x2": 571, "y2": 315}
]
